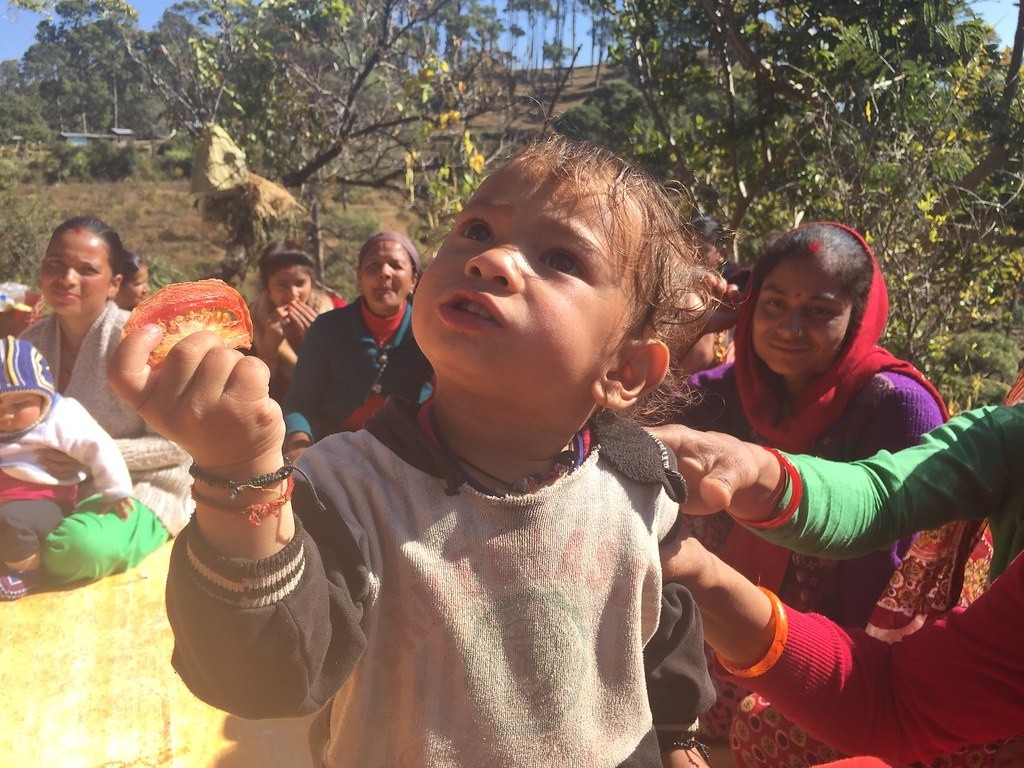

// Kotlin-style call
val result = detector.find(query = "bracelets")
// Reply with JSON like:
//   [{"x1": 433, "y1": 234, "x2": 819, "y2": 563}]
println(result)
[
  {"x1": 726, "y1": 446, "x2": 803, "y2": 528},
  {"x1": 185, "y1": 453, "x2": 332, "y2": 514},
  {"x1": 714, "y1": 582, "x2": 790, "y2": 679},
  {"x1": 188, "y1": 462, "x2": 298, "y2": 527},
  {"x1": 658, "y1": 738, "x2": 714, "y2": 767}
]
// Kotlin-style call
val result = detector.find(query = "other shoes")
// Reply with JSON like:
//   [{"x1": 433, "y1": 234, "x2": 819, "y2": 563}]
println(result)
[{"x1": 0, "y1": 564, "x2": 46, "y2": 600}]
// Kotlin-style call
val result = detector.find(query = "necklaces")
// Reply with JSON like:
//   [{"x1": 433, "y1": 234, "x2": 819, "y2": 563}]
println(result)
[{"x1": 441, "y1": 434, "x2": 587, "y2": 490}]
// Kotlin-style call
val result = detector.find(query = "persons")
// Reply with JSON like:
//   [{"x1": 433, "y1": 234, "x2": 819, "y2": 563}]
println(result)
[{"x1": 0, "y1": 141, "x2": 1024, "y2": 768}]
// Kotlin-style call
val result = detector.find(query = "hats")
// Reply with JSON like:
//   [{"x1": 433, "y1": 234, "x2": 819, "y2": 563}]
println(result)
[{"x1": 0, "y1": 335, "x2": 60, "y2": 440}]
